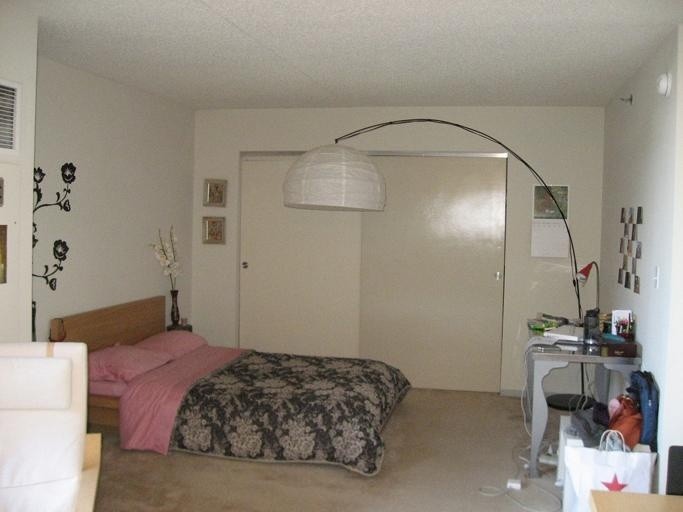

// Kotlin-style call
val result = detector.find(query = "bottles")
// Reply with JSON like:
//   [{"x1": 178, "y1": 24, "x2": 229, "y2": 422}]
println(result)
[{"x1": 582, "y1": 307, "x2": 600, "y2": 346}]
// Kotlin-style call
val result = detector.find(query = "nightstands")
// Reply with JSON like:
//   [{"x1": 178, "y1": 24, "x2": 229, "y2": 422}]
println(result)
[{"x1": 167, "y1": 324, "x2": 192, "y2": 333}]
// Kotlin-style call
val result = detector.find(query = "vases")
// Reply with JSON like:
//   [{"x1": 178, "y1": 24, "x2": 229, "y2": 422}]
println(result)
[{"x1": 170, "y1": 289, "x2": 180, "y2": 325}]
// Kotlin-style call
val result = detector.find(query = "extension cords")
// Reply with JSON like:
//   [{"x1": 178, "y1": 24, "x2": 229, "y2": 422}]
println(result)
[{"x1": 538, "y1": 452, "x2": 558, "y2": 467}]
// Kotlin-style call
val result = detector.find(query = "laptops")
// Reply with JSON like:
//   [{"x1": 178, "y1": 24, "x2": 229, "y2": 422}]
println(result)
[{"x1": 543, "y1": 324, "x2": 584, "y2": 341}]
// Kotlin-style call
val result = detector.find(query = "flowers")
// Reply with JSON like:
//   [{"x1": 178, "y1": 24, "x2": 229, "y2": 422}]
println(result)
[{"x1": 149, "y1": 224, "x2": 182, "y2": 289}]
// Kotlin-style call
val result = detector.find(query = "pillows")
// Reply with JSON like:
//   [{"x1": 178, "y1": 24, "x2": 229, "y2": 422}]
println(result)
[
  {"x1": 133, "y1": 330, "x2": 209, "y2": 362},
  {"x1": 88, "y1": 346, "x2": 176, "y2": 383}
]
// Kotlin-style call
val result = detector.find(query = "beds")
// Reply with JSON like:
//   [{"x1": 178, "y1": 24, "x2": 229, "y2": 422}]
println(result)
[{"x1": 50, "y1": 294, "x2": 411, "y2": 476}]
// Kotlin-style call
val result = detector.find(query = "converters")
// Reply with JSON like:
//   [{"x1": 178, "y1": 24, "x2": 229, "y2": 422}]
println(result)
[{"x1": 507, "y1": 477, "x2": 523, "y2": 490}]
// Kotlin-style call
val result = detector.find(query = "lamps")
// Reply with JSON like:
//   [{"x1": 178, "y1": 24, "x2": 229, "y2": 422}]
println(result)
[
  {"x1": 655, "y1": 70, "x2": 672, "y2": 98},
  {"x1": 577, "y1": 261, "x2": 600, "y2": 312},
  {"x1": 284, "y1": 119, "x2": 596, "y2": 410}
]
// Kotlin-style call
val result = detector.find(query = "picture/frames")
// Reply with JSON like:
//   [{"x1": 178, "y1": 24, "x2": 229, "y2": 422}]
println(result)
[
  {"x1": 203, "y1": 178, "x2": 228, "y2": 208},
  {"x1": 0, "y1": 225, "x2": 7, "y2": 284},
  {"x1": 202, "y1": 216, "x2": 226, "y2": 245}
]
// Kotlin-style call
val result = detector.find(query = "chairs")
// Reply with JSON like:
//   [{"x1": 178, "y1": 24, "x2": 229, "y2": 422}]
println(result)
[{"x1": 0, "y1": 339, "x2": 102, "y2": 512}]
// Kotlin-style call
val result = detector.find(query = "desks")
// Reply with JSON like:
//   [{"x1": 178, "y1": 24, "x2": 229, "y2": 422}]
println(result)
[
  {"x1": 589, "y1": 490, "x2": 683, "y2": 512},
  {"x1": 527, "y1": 320, "x2": 642, "y2": 479}
]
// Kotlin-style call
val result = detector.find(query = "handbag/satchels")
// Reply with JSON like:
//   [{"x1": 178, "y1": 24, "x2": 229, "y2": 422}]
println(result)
[
  {"x1": 562, "y1": 430, "x2": 657, "y2": 512},
  {"x1": 555, "y1": 370, "x2": 659, "y2": 487}
]
